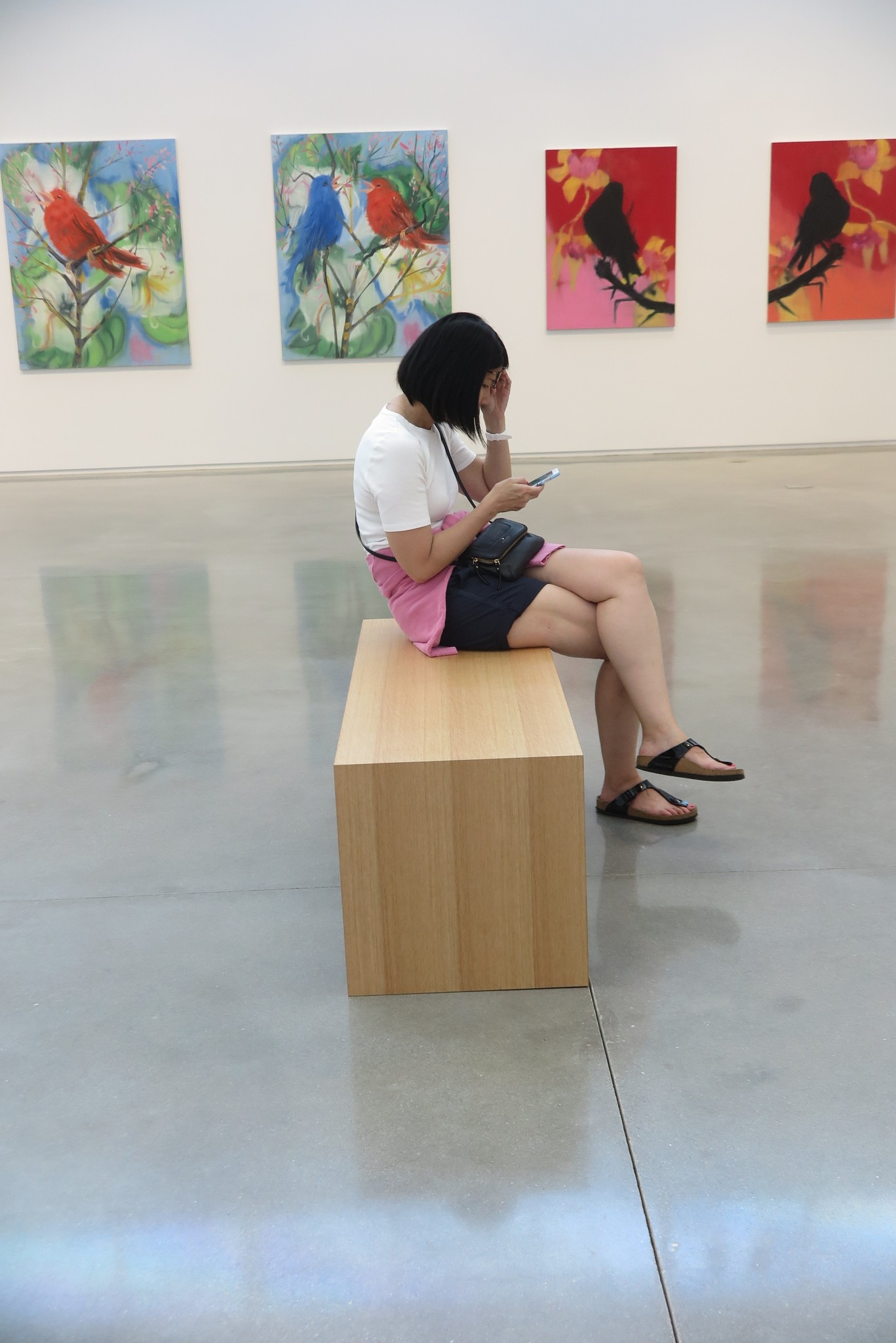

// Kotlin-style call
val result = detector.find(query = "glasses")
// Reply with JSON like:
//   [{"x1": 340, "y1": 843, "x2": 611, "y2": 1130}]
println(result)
[{"x1": 486, "y1": 370, "x2": 502, "y2": 389}]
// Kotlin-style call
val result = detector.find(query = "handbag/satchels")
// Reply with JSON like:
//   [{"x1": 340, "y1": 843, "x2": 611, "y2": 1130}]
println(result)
[{"x1": 458, "y1": 518, "x2": 545, "y2": 590}]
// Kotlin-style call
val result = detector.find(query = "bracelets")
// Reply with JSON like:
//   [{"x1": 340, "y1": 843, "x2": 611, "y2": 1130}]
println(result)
[{"x1": 485, "y1": 426, "x2": 512, "y2": 441}]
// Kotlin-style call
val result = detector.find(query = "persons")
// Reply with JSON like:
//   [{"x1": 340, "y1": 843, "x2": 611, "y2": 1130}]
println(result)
[{"x1": 352, "y1": 312, "x2": 745, "y2": 827}]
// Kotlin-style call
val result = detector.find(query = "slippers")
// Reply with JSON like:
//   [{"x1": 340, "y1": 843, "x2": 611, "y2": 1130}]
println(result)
[
  {"x1": 594, "y1": 779, "x2": 698, "y2": 825},
  {"x1": 637, "y1": 736, "x2": 745, "y2": 782}
]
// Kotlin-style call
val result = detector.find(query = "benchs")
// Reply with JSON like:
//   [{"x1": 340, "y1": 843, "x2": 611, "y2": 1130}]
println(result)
[{"x1": 334, "y1": 618, "x2": 590, "y2": 997}]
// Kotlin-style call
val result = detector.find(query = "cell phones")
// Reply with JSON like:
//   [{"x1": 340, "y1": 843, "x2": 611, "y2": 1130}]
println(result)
[{"x1": 527, "y1": 469, "x2": 559, "y2": 487}]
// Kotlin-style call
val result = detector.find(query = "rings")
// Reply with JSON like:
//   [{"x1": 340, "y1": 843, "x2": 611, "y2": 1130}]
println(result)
[{"x1": 500, "y1": 380, "x2": 504, "y2": 383}]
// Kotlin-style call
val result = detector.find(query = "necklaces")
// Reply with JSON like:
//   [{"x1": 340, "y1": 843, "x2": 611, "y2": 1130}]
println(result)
[{"x1": 388, "y1": 403, "x2": 436, "y2": 434}]
